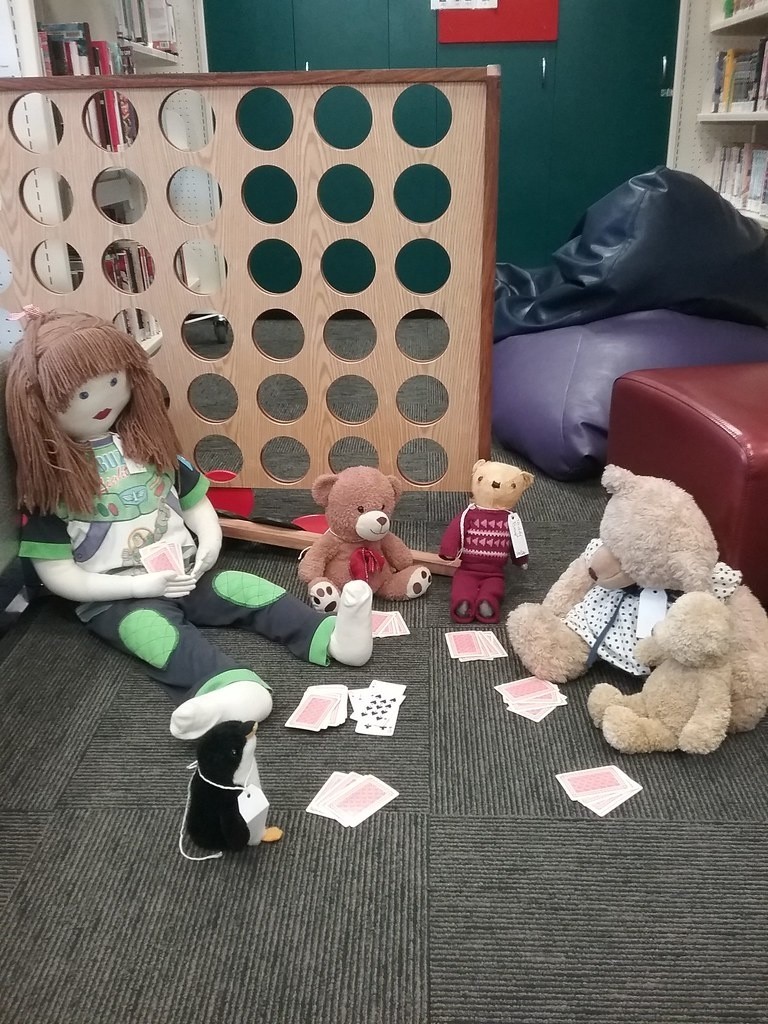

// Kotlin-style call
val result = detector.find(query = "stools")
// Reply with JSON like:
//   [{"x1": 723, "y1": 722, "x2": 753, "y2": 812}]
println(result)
[{"x1": 608, "y1": 362, "x2": 768, "y2": 613}]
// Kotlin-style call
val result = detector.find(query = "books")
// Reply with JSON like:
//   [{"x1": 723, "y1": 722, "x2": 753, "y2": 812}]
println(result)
[{"x1": 36, "y1": 1, "x2": 179, "y2": 344}]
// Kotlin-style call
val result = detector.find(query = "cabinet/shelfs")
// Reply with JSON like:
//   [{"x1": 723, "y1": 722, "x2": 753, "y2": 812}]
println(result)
[
  {"x1": 0, "y1": 0, "x2": 232, "y2": 357},
  {"x1": 665, "y1": 0, "x2": 768, "y2": 234}
]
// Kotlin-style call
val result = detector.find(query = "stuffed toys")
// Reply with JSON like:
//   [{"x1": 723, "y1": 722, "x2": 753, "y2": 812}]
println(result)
[
  {"x1": 3, "y1": 305, "x2": 374, "y2": 740},
  {"x1": 509, "y1": 464, "x2": 767, "y2": 732},
  {"x1": 439, "y1": 458, "x2": 535, "y2": 624},
  {"x1": 298, "y1": 460, "x2": 431, "y2": 614},
  {"x1": 586, "y1": 592, "x2": 730, "y2": 755},
  {"x1": 190, "y1": 719, "x2": 271, "y2": 845}
]
